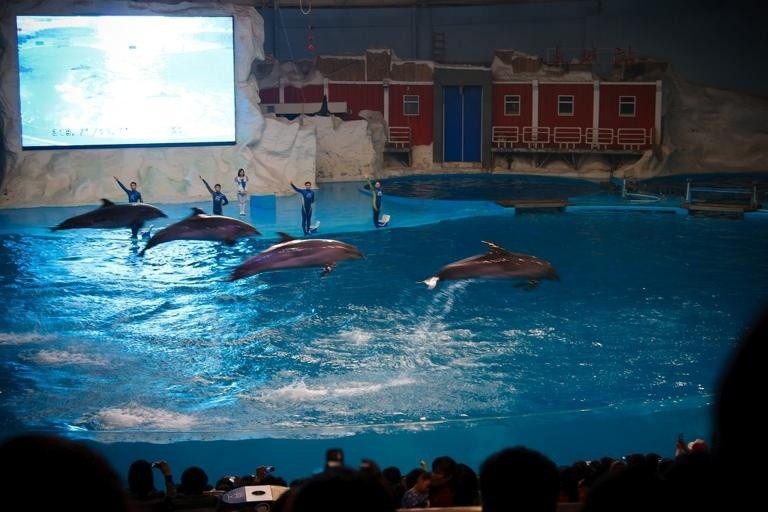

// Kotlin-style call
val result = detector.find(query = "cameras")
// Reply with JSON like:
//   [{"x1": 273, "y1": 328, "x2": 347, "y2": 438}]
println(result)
[
  {"x1": 265, "y1": 466, "x2": 275, "y2": 473},
  {"x1": 152, "y1": 461, "x2": 162, "y2": 468}
]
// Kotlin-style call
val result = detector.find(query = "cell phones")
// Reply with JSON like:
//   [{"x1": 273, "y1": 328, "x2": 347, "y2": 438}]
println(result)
[{"x1": 325, "y1": 448, "x2": 343, "y2": 471}]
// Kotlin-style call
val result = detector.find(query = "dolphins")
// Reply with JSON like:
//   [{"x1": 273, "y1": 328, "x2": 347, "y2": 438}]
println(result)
[
  {"x1": 413, "y1": 239, "x2": 560, "y2": 291},
  {"x1": 48, "y1": 198, "x2": 168, "y2": 238},
  {"x1": 137, "y1": 206, "x2": 262, "y2": 257},
  {"x1": 214, "y1": 231, "x2": 367, "y2": 283}
]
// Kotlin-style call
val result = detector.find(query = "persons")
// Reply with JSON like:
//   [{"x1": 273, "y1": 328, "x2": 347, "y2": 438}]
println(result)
[
  {"x1": 174, "y1": 466, "x2": 221, "y2": 511},
  {"x1": 288, "y1": 176, "x2": 315, "y2": 236},
  {"x1": 233, "y1": 168, "x2": 250, "y2": 216},
  {"x1": 362, "y1": 171, "x2": 386, "y2": 229},
  {"x1": 1, "y1": 435, "x2": 124, "y2": 511},
  {"x1": 111, "y1": 172, "x2": 144, "y2": 241},
  {"x1": 196, "y1": 172, "x2": 228, "y2": 216},
  {"x1": 215, "y1": 465, "x2": 289, "y2": 510},
  {"x1": 124, "y1": 460, "x2": 178, "y2": 510},
  {"x1": 274, "y1": 441, "x2": 712, "y2": 511}
]
